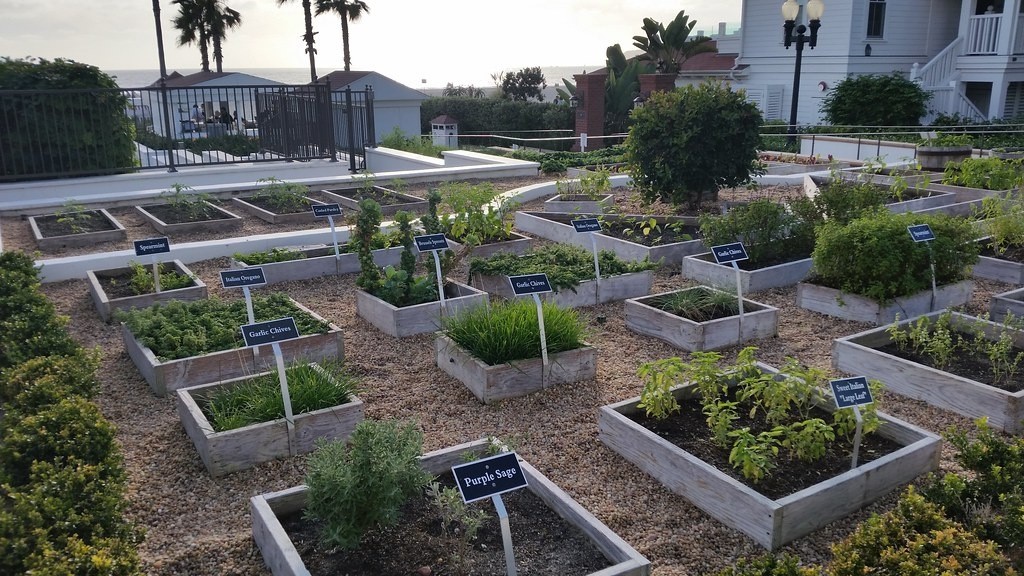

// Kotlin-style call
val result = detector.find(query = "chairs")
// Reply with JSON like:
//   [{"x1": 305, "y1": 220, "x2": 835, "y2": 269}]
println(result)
[{"x1": 207, "y1": 123, "x2": 227, "y2": 138}]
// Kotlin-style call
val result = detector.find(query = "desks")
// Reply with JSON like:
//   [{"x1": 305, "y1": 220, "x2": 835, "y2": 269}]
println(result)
[{"x1": 182, "y1": 128, "x2": 296, "y2": 148}]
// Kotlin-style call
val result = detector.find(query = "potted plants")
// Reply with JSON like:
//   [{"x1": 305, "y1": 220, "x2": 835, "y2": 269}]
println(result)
[
  {"x1": 543, "y1": 163, "x2": 615, "y2": 214},
  {"x1": 629, "y1": 283, "x2": 782, "y2": 354},
  {"x1": 916, "y1": 130, "x2": 973, "y2": 173},
  {"x1": 233, "y1": 175, "x2": 343, "y2": 224},
  {"x1": 943, "y1": 191, "x2": 1024, "y2": 284},
  {"x1": 681, "y1": 187, "x2": 879, "y2": 295},
  {"x1": 803, "y1": 153, "x2": 956, "y2": 225},
  {"x1": 230, "y1": 228, "x2": 445, "y2": 282},
  {"x1": 596, "y1": 349, "x2": 942, "y2": 548},
  {"x1": 835, "y1": 166, "x2": 946, "y2": 186},
  {"x1": 921, "y1": 147, "x2": 1024, "y2": 200},
  {"x1": 29, "y1": 198, "x2": 126, "y2": 249},
  {"x1": 414, "y1": 179, "x2": 533, "y2": 270},
  {"x1": 247, "y1": 433, "x2": 652, "y2": 576},
  {"x1": 515, "y1": 210, "x2": 800, "y2": 268},
  {"x1": 171, "y1": 361, "x2": 362, "y2": 474},
  {"x1": 830, "y1": 309, "x2": 1024, "y2": 435},
  {"x1": 86, "y1": 259, "x2": 207, "y2": 319},
  {"x1": 427, "y1": 272, "x2": 598, "y2": 407},
  {"x1": 989, "y1": 147, "x2": 1024, "y2": 161},
  {"x1": 320, "y1": 169, "x2": 430, "y2": 215},
  {"x1": 343, "y1": 218, "x2": 489, "y2": 341},
  {"x1": 625, "y1": 284, "x2": 780, "y2": 353},
  {"x1": 468, "y1": 242, "x2": 654, "y2": 314},
  {"x1": 114, "y1": 294, "x2": 345, "y2": 395},
  {"x1": 135, "y1": 183, "x2": 242, "y2": 235}
]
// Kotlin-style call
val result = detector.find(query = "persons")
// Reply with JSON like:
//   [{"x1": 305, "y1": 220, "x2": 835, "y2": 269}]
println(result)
[{"x1": 192, "y1": 103, "x2": 256, "y2": 128}]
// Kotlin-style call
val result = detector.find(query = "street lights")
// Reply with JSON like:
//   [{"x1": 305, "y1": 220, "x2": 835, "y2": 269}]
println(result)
[{"x1": 782, "y1": 0, "x2": 824, "y2": 154}]
